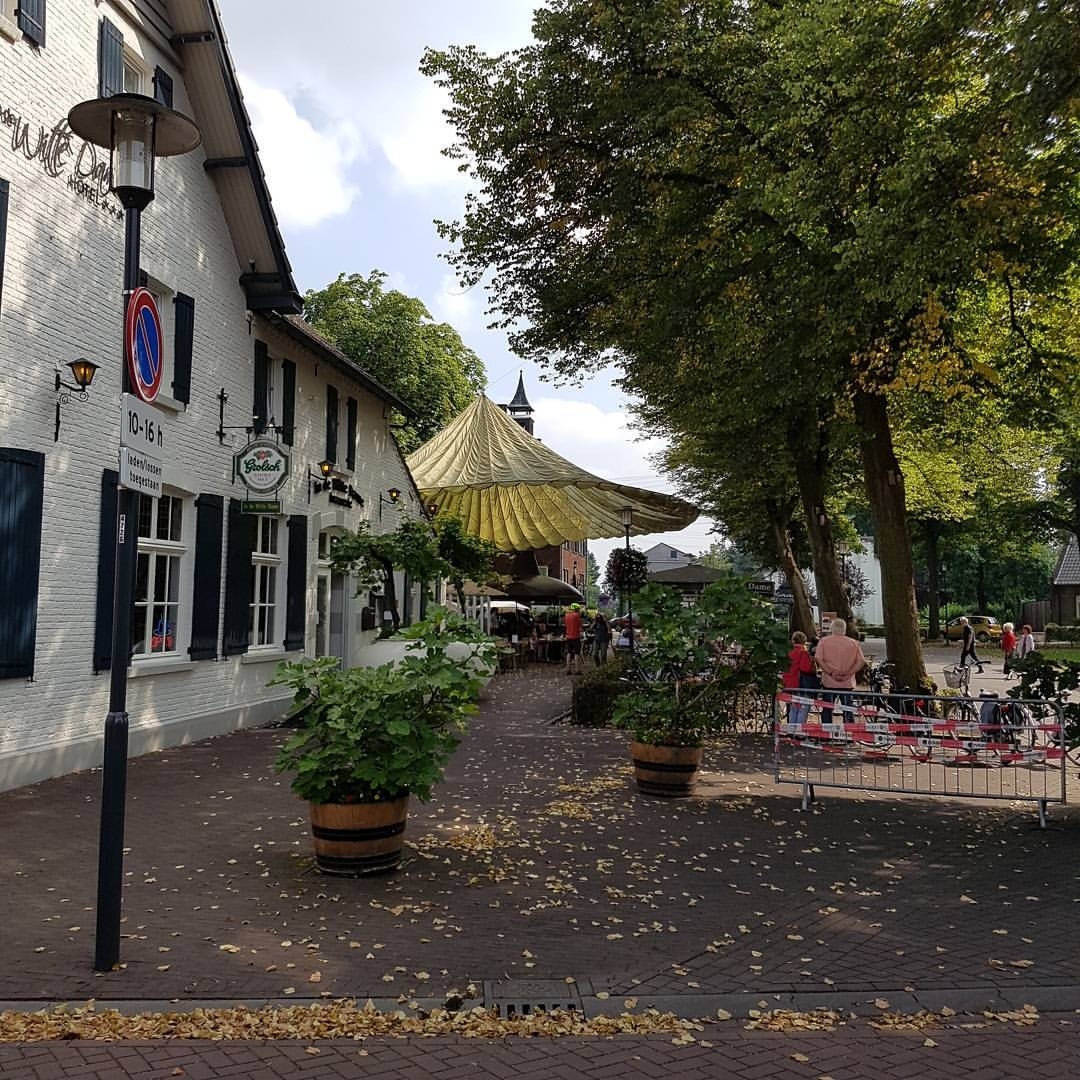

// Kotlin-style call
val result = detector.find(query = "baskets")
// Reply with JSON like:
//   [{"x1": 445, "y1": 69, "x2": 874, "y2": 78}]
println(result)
[{"x1": 943, "y1": 663, "x2": 971, "y2": 688}]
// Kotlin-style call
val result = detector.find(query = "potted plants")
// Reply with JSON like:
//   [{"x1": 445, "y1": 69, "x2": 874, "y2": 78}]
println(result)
[
  {"x1": 264, "y1": 600, "x2": 514, "y2": 877},
  {"x1": 609, "y1": 692, "x2": 728, "y2": 797}
]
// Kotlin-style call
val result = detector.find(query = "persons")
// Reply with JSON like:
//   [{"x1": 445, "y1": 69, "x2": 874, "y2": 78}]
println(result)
[
  {"x1": 479, "y1": 604, "x2": 616, "y2": 674},
  {"x1": 784, "y1": 617, "x2": 1032, "y2": 740}
]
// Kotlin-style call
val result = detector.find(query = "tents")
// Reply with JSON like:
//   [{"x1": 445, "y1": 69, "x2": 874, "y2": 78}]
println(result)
[{"x1": 403, "y1": 390, "x2": 727, "y2": 628}]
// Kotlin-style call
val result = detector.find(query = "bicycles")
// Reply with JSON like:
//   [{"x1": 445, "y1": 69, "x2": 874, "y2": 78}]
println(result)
[
  {"x1": 851, "y1": 660, "x2": 934, "y2": 762},
  {"x1": 942, "y1": 660, "x2": 1080, "y2": 767}
]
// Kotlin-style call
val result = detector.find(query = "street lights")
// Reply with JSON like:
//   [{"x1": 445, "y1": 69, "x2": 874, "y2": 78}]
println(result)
[
  {"x1": 682, "y1": 553, "x2": 696, "y2": 609},
  {"x1": 67, "y1": 89, "x2": 201, "y2": 973},
  {"x1": 835, "y1": 540, "x2": 851, "y2": 586},
  {"x1": 612, "y1": 506, "x2": 640, "y2": 661}
]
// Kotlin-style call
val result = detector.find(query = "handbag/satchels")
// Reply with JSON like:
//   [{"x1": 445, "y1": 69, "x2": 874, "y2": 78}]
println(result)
[{"x1": 799, "y1": 671, "x2": 823, "y2": 700}]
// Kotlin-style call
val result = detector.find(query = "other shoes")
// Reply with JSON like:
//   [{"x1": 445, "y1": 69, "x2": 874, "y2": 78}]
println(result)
[
  {"x1": 575, "y1": 671, "x2": 583, "y2": 675},
  {"x1": 566, "y1": 671, "x2": 572, "y2": 675},
  {"x1": 975, "y1": 669, "x2": 983, "y2": 673}
]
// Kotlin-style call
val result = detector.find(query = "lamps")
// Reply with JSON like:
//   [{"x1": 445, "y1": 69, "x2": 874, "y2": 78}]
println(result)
[
  {"x1": 307, "y1": 460, "x2": 334, "y2": 481},
  {"x1": 419, "y1": 503, "x2": 438, "y2": 517},
  {"x1": 380, "y1": 488, "x2": 401, "y2": 504},
  {"x1": 55, "y1": 358, "x2": 101, "y2": 404}
]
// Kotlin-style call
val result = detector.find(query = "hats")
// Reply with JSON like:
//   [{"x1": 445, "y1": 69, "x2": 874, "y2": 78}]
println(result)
[{"x1": 571, "y1": 603, "x2": 581, "y2": 609}]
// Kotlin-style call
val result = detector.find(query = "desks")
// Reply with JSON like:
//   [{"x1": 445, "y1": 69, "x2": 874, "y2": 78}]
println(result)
[{"x1": 523, "y1": 634, "x2": 567, "y2": 663}]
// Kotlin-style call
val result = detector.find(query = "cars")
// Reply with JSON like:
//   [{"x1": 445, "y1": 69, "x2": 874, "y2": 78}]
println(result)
[
  {"x1": 937, "y1": 616, "x2": 1006, "y2": 642},
  {"x1": 608, "y1": 611, "x2": 661, "y2": 633}
]
